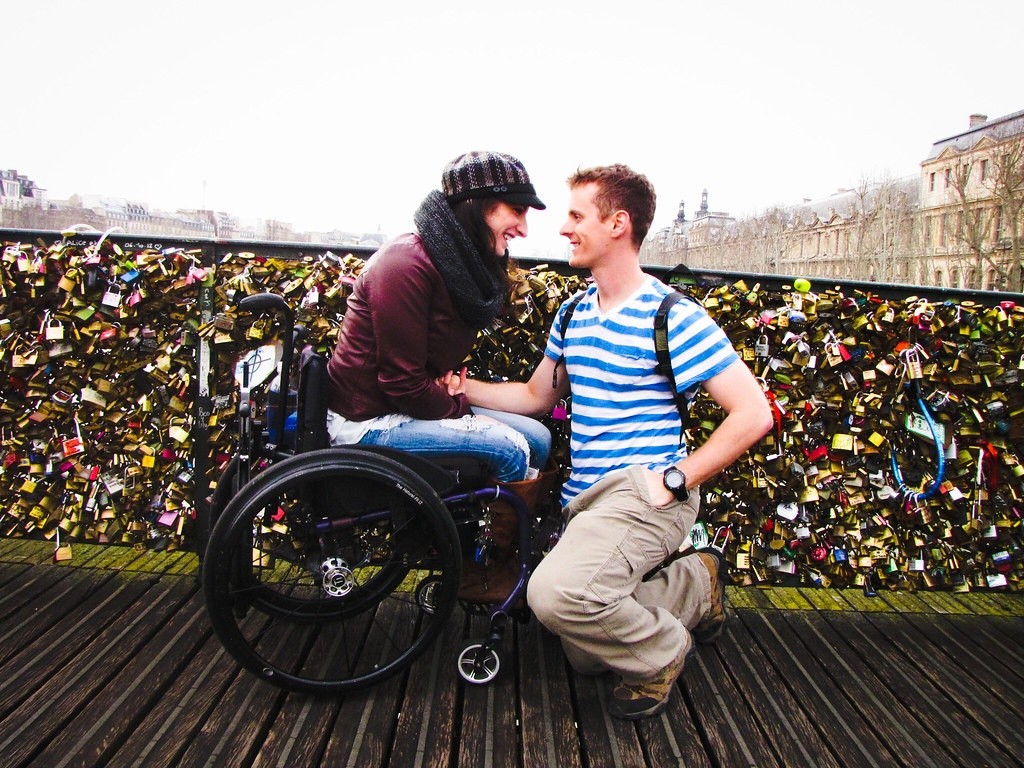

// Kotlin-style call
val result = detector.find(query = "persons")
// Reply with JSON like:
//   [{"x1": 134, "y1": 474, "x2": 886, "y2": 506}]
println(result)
[
  {"x1": 435, "y1": 166, "x2": 775, "y2": 722},
  {"x1": 323, "y1": 149, "x2": 554, "y2": 606}
]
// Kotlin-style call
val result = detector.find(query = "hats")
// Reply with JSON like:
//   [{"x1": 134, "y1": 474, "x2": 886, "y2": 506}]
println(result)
[{"x1": 441, "y1": 151, "x2": 546, "y2": 210}]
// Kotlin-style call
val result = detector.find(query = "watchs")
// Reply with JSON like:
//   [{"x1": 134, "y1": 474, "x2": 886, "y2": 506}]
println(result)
[{"x1": 663, "y1": 466, "x2": 690, "y2": 501}]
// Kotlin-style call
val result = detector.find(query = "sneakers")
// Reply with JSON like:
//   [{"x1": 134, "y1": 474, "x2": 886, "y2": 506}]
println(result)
[
  {"x1": 692, "y1": 548, "x2": 729, "y2": 648},
  {"x1": 608, "y1": 654, "x2": 686, "y2": 718}
]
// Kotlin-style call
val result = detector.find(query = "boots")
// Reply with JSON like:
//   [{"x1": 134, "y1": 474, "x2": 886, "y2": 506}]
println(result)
[{"x1": 456, "y1": 454, "x2": 559, "y2": 613}]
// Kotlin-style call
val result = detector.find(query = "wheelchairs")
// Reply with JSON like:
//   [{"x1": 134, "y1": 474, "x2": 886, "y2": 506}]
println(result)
[{"x1": 198, "y1": 291, "x2": 565, "y2": 699}]
